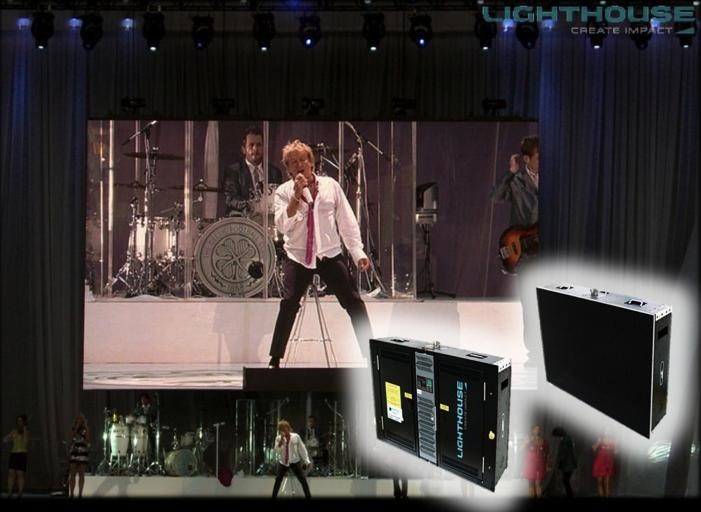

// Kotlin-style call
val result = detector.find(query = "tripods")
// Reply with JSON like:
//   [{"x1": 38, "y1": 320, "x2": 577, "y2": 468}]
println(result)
[
  {"x1": 283, "y1": 280, "x2": 338, "y2": 369},
  {"x1": 95, "y1": 418, "x2": 165, "y2": 475},
  {"x1": 418, "y1": 227, "x2": 456, "y2": 299},
  {"x1": 279, "y1": 469, "x2": 303, "y2": 498},
  {"x1": 347, "y1": 168, "x2": 388, "y2": 297},
  {"x1": 102, "y1": 166, "x2": 206, "y2": 300}
]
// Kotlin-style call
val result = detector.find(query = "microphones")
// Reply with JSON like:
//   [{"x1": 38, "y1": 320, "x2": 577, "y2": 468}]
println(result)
[
  {"x1": 364, "y1": 137, "x2": 385, "y2": 157},
  {"x1": 301, "y1": 178, "x2": 313, "y2": 205},
  {"x1": 128, "y1": 119, "x2": 162, "y2": 142},
  {"x1": 210, "y1": 421, "x2": 227, "y2": 427}
]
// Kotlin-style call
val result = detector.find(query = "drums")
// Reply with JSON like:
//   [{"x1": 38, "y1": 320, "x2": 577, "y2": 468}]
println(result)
[
  {"x1": 111, "y1": 424, "x2": 130, "y2": 456},
  {"x1": 132, "y1": 424, "x2": 147, "y2": 458},
  {"x1": 128, "y1": 217, "x2": 176, "y2": 260},
  {"x1": 177, "y1": 217, "x2": 219, "y2": 257},
  {"x1": 180, "y1": 432, "x2": 195, "y2": 447},
  {"x1": 165, "y1": 451, "x2": 197, "y2": 476},
  {"x1": 193, "y1": 217, "x2": 277, "y2": 298}
]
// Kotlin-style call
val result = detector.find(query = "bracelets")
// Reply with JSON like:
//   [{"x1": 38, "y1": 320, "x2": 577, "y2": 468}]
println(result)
[{"x1": 293, "y1": 193, "x2": 299, "y2": 202}]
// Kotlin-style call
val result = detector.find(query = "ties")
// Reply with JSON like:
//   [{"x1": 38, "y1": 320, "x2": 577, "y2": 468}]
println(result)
[
  {"x1": 253, "y1": 166, "x2": 260, "y2": 184},
  {"x1": 284, "y1": 438, "x2": 291, "y2": 464},
  {"x1": 301, "y1": 187, "x2": 319, "y2": 265}
]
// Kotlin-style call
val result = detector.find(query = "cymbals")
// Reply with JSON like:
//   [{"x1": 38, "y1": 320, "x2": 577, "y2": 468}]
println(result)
[
  {"x1": 170, "y1": 184, "x2": 221, "y2": 193},
  {"x1": 124, "y1": 151, "x2": 185, "y2": 160},
  {"x1": 116, "y1": 182, "x2": 166, "y2": 193}
]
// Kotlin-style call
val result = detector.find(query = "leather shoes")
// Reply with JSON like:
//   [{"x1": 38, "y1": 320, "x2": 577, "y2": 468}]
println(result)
[{"x1": 269, "y1": 361, "x2": 280, "y2": 368}]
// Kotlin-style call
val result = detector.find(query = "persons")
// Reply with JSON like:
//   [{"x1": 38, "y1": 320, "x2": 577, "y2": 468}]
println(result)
[
  {"x1": 591, "y1": 436, "x2": 617, "y2": 497},
  {"x1": 139, "y1": 395, "x2": 155, "y2": 414},
  {"x1": 68, "y1": 413, "x2": 90, "y2": 498},
  {"x1": 553, "y1": 427, "x2": 578, "y2": 495},
  {"x1": 268, "y1": 139, "x2": 372, "y2": 368},
  {"x1": 490, "y1": 135, "x2": 535, "y2": 368},
  {"x1": 272, "y1": 420, "x2": 312, "y2": 498},
  {"x1": 524, "y1": 425, "x2": 551, "y2": 498},
  {"x1": 393, "y1": 456, "x2": 408, "y2": 501},
  {"x1": 300, "y1": 415, "x2": 321, "y2": 441},
  {"x1": 222, "y1": 129, "x2": 282, "y2": 216},
  {"x1": 3, "y1": 415, "x2": 31, "y2": 498}
]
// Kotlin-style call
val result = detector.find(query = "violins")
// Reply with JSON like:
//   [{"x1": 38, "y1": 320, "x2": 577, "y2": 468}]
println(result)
[{"x1": 19, "y1": 423, "x2": 31, "y2": 432}]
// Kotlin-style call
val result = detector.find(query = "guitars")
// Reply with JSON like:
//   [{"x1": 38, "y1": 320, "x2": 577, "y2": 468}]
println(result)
[{"x1": 498, "y1": 220, "x2": 541, "y2": 274}]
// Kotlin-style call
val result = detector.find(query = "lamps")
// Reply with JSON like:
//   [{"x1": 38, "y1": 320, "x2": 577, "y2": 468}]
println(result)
[{"x1": 30, "y1": 1, "x2": 701, "y2": 54}]
